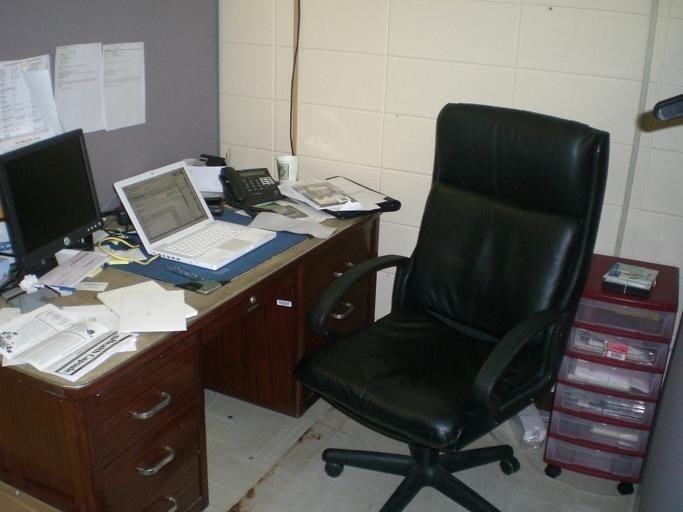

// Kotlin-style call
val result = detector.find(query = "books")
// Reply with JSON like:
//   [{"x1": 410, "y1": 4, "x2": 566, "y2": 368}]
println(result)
[{"x1": 277, "y1": 173, "x2": 401, "y2": 218}]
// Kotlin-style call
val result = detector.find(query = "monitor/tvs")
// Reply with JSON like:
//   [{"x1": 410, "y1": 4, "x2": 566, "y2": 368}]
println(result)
[{"x1": 0, "y1": 128, "x2": 104, "y2": 279}]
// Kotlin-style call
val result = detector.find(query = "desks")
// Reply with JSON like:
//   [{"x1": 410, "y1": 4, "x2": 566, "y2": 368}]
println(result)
[{"x1": 0, "y1": 198, "x2": 381, "y2": 512}]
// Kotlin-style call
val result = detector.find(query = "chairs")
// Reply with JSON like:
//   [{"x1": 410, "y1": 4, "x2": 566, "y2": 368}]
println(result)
[{"x1": 294, "y1": 101, "x2": 611, "y2": 512}]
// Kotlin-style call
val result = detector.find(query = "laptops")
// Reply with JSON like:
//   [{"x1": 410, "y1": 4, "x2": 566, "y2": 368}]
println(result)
[{"x1": 112, "y1": 160, "x2": 278, "y2": 271}]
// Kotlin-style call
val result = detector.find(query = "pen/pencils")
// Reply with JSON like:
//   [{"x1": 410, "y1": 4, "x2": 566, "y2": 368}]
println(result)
[{"x1": 166, "y1": 262, "x2": 202, "y2": 282}]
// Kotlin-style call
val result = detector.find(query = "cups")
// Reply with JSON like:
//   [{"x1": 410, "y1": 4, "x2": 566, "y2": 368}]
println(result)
[{"x1": 274, "y1": 156, "x2": 298, "y2": 184}]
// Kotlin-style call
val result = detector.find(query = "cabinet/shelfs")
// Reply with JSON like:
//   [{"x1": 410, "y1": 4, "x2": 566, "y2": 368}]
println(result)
[{"x1": 541, "y1": 251, "x2": 680, "y2": 494}]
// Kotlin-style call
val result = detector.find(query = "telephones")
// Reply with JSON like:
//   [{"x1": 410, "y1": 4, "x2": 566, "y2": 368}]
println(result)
[{"x1": 218, "y1": 167, "x2": 281, "y2": 206}]
